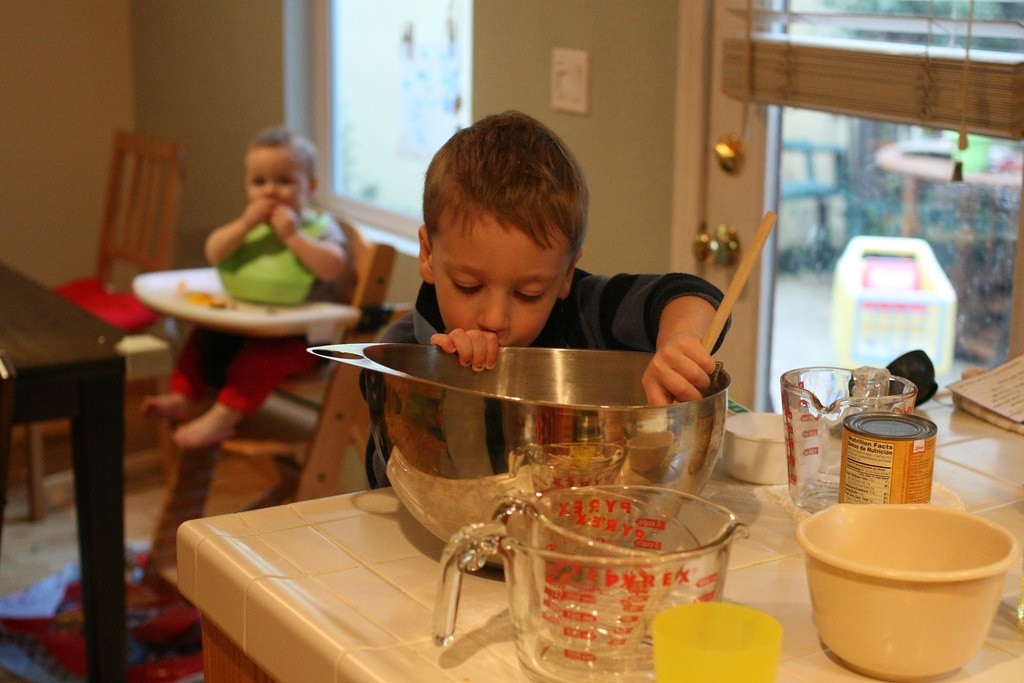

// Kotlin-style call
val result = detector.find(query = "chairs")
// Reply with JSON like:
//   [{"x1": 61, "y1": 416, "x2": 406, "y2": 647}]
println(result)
[
  {"x1": 7, "y1": 127, "x2": 186, "y2": 526},
  {"x1": 135, "y1": 212, "x2": 419, "y2": 568}
]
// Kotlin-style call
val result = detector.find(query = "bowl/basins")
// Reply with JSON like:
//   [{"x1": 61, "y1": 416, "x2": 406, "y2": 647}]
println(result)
[
  {"x1": 722, "y1": 412, "x2": 820, "y2": 484},
  {"x1": 796, "y1": 502, "x2": 1018, "y2": 683},
  {"x1": 306, "y1": 337, "x2": 729, "y2": 566}
]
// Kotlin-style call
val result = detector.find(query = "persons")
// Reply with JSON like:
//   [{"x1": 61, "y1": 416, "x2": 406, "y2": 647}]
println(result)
[
  {"x1": 136, "y1": 126, "x2": 349, "y2": 449},
  {"x1": 358, "y1": 108, "x2": 734, "y2": 489}
]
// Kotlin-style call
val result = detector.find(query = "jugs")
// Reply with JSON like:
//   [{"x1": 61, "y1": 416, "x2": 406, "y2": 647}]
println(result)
[
  {"x1": 780, "y1": 366, "x2": 918, "y2": 514},
  {"x1": 433, "y1": 483, "x2": 748, "y2": 682}
]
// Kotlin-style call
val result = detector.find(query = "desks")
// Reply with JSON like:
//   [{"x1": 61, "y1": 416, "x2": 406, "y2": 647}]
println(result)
[{"x1": 0, "y1": 258, "x2": 174, "y2": 683}]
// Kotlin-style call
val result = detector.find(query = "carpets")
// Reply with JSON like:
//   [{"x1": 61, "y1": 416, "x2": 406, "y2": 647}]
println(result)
[{"x1": 1, "y1": 541, "x2": 208, "y2": 683}]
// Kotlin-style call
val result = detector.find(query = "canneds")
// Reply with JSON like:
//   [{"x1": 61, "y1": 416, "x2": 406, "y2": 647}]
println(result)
[{"x1": 839, "y1": 410, "x2": 939, "y2": 506}]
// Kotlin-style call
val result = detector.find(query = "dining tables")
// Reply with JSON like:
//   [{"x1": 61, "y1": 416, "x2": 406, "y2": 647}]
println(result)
[{"x1": 169, "y1": 392, "x2": 1024, "y2": 683}]
